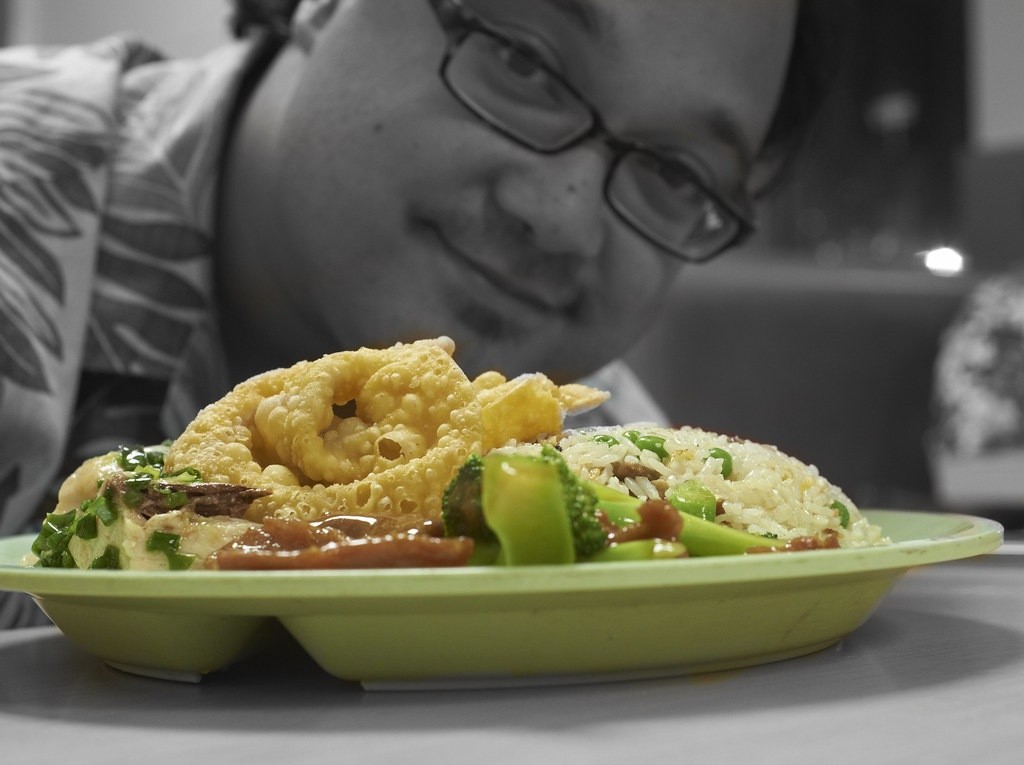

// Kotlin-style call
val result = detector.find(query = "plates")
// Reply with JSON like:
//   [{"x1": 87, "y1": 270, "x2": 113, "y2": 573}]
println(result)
[{"x1": 1, "y1": 510, "x2": 1004, "y2": 692}]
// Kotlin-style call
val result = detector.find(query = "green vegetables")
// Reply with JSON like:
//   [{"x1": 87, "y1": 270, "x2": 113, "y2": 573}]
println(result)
[{"x1": 30, "y1": 448, "x2": 203, "y2": 570}]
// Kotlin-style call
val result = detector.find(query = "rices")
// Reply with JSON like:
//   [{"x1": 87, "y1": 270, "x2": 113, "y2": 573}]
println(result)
[{"x1": 557, "y1": 424, "x2": 893, "y2": 548}]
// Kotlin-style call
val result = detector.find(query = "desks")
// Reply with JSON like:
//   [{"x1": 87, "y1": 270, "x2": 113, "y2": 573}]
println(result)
[{"x1": 0, "y1": 555, "x2": 1024, "y2": 765}]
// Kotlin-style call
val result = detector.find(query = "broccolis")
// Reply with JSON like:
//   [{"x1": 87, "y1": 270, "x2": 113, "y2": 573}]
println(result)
[{"x1": 440, "y1": 444, "x2": 798, "y2": 566}]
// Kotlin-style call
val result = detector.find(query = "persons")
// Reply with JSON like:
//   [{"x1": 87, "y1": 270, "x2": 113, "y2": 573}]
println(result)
[{"x1": 0, "y1": 1, "x2": 825, "y2": 632}]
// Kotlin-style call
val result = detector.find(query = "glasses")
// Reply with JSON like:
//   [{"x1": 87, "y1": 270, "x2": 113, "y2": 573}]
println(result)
[{"x1": 429, "y1": 0, "x2": 759, "y2": 265}]
